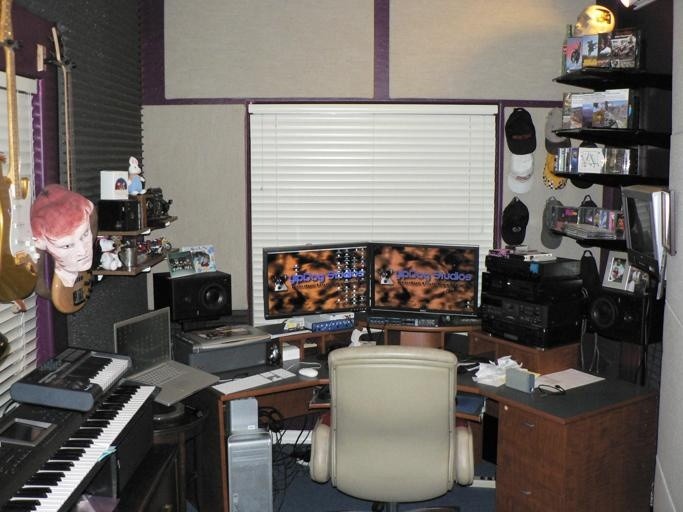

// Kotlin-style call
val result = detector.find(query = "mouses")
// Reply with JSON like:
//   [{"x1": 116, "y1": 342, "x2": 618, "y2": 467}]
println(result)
[{"x1": 298, "y1": 368, "x2": 319, "y2": 378}]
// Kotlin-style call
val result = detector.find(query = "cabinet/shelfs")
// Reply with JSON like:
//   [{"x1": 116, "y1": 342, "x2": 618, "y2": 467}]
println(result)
[
  {"x1": 551, "y1": 0, "x2": 673, "y2": 188},
  {"x1": 116, "y1": 400, "x2": 150, "y2": 494}
]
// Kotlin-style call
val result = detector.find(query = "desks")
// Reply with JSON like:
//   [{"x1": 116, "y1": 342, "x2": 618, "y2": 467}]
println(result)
[
  {"x1": 181, "y1": 318, "x2": 659, "y2": 512},
  {"x1": 150, "y1": 396, "x2": 212, "y2": 512}
]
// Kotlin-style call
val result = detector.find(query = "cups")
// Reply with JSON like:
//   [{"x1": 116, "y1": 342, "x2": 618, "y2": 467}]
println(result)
[{"x1": 117, "y1": 245, "x2": 137, "y2": 268}]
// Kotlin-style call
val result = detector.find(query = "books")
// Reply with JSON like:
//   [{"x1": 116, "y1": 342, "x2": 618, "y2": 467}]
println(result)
[
  {"x1": 562, "y1": 31, "x2": 641, "y2": 75},
  {"x1": 553, "y1": 207, "x2": 625, "y2": 242},
  {"x1": 561, "y1": 89, "x2": 630, "y2": 130},
  {"x1": 555, "y1": 147, "x2": 637, "y2": 175}
]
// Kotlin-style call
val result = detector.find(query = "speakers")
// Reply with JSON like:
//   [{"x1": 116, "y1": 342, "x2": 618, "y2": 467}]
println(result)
[
  {"x1": 154, "y1": 270, "x2": 232, "y2": 323},
  {"x1": 585, "y1": 286, "x2": 664, "y2": 343}
]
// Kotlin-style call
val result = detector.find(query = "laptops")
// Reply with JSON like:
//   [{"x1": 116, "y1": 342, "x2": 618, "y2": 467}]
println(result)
[{"x1": 114, "y1": 307, "x2": 219, "y2": 408}]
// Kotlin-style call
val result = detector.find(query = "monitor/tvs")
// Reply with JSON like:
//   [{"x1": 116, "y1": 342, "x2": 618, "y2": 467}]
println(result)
[
  {"x1": 262, "y1": 242, "x2": 372, "y2": 330},
  {"x1": 620, "y1": 186, "x2": 663, "y2": 283},
  {"x1": 372, "y1": 242, "x2": 480, "y2": 317}
]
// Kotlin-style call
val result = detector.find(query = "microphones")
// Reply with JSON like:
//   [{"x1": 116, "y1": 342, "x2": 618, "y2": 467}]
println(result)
[{"x1": 538, "y1": 384, "x2": 567, "y2": 396}]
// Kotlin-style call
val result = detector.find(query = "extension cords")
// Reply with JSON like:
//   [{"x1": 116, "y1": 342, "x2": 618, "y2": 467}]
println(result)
[
  {"x1": 472, "y1": 477, "x2": 497, "y2": 489},
  {"x1": 296, "y1": 458, "x2": 309, "y2": 466}
]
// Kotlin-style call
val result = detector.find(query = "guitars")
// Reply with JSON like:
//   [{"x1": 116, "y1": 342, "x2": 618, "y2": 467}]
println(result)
[
  {"x1": 40, "y1": 27, "x2": 93, "y2": 314},
  {"x1": 0, "y1": 0, "x2": 36, "y2": 303}
]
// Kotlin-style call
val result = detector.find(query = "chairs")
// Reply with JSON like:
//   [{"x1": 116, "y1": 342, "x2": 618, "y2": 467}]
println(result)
[{"x1": 311, "y1": 346, "x2": 475, "y2": 511}]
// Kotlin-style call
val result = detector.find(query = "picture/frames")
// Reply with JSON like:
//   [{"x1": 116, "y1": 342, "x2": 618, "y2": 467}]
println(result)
[
  {"x1": 183, "y1": 244, "x2": 217, "y2": 272},
  {"x1": 601, "y1": 251, "x2": 630, "y2": 292},
  {"x1": 167, "y1": 251, "x2": 197, "y2": 278}
]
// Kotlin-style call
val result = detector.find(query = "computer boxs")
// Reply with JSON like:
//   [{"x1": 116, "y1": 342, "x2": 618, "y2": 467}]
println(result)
[{"x1": 228, "y1": 433, "x2": 272, "y2": 512}]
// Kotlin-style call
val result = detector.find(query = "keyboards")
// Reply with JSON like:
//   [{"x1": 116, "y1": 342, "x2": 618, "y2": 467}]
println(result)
[
  {"x1": 317, "y1": 385, "x2": 330, "y2": 401},
  {"x1": 211, "y1": 369, "x2": 296, "y2": 395}
]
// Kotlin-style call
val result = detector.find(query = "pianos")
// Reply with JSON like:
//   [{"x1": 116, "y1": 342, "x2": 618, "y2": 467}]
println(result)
[
  {"x1": 10, "y1": 345, "x2": 132, "y2": 411},
  {"x1": 0, "y1": 378, "x2": 162, "y2": 512}
]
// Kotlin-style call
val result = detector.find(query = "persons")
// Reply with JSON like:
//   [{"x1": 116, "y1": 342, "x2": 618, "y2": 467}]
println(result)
[
  {"x1": 614, "y1": 259, "x2": 624, "y2": 278},
  {"x1": 633, "y1": 267, "x2": 642, "y2": 292},
  {"x1": 30, "y1": 185, "x2": 92, "y2": 287},
  {"x1": 609, "y1": 269, "x2": 621, "y2": 283}
]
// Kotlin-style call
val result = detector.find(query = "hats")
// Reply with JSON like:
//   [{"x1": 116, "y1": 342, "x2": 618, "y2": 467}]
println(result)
[
  {"x1": 580, "y1": 250, "x2": 601, "y2": 293},
  {"x1": 508, "y1": 153, "x2": 534, "y2": 193},
  {"x1": 505, "y1": 108, "x2": 536, "y2": 155},
  {"x1": 576, "y1": 194, "x2": 600, "y2": 248},
  {"x1": 545, "y1": 107, "x2": 571, "y2": 155},
  {"x1": 543, "y1": 153, "x2": 569, "y2": 190},
  {"x1": 571, "y1": 141, "x2": 599, "y2": 188},
  {"x1": 501, "y1": 197, "x2": 529, "y2": 245},
  {"x1": 540, "y1": 196, "x2": 564, "y2": 249}
]
